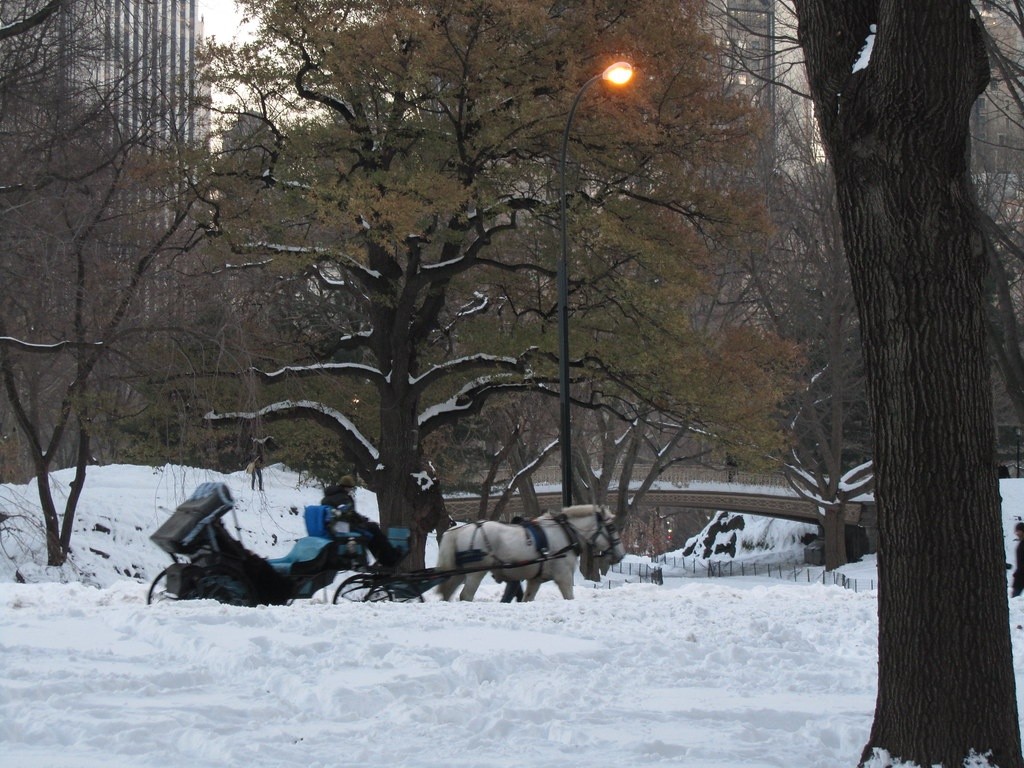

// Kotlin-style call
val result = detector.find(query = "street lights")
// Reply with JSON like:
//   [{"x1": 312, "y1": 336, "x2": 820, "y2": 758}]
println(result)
[{"x1": 559, "y1": 60, "x2": 634, "y2": 507}]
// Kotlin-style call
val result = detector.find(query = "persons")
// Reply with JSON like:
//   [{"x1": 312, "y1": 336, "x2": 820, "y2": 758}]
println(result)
[
  {"x1": 246, "y1": 443, "x2": 265, "y2": 492},
  {"x1": 724, "y1": 452, "x2": 737, "y2": 485},
  {"x1": 318, "y1": 476, "x2": 406, "y2": 570},
  {"x1": 1009, "y1": 519, "x2": 1024, "y2": 597},
  {"x1": 997, "y1": 456, "x2": 1011, "y2": 478}
]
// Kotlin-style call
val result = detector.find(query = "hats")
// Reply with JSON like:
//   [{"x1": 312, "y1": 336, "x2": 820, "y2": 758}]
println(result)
[{"x1": 338, "y1": 475, "x2": 355, "y2": 487}]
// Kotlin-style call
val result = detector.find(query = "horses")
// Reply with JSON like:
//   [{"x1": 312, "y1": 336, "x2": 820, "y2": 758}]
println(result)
[{"x1": 437, "y1": 504, "x2": 625, "y2": 603}]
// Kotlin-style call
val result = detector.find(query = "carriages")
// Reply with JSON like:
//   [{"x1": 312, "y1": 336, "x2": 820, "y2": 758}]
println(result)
[{"x1": 147, "y1": 481, "x2": 627, "y2": 607}]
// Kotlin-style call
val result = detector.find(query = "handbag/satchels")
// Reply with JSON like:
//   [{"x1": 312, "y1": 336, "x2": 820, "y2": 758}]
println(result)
[{"x1": 245, "y1": 462, "x2": 255, "y2": 475}]
